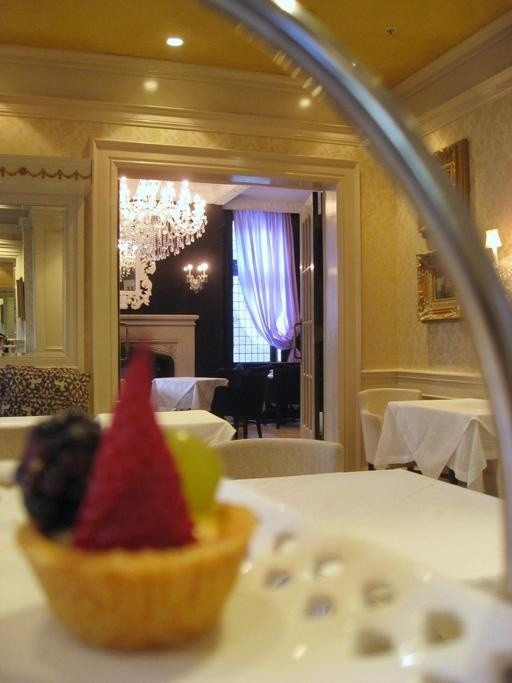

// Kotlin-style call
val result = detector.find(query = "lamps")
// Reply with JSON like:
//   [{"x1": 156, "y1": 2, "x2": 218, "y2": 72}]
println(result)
[
  {"x1": 118, "y1": 176, "x2": 208, "y2": 265},
  {"x1": 184, "y1": 261, "x2": 208, "y2": 291}
]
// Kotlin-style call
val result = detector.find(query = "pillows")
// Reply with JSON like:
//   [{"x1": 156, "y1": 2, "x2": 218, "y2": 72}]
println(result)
[{"x1": 0, "y1": 362, "x2": 91, "y2": 416}]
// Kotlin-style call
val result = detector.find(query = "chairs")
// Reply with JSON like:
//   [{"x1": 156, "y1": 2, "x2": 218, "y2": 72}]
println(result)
[
  {"x1": 119, "y1": 340, "x2": 300, "y2": 440},
  {"x1": 358, "y1": 386, "x2": 424, "y2": 473},
  {"x1": 207, "y1": 435, "x2": 345, "y2": 481},
  {"x1": 1, "y1": 422, "x2": 40, "y2": 459}
]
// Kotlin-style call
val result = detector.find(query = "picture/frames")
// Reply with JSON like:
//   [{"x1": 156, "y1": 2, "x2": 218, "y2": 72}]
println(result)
[
  {"x1": 413, "y1": 250, "x2": 493, "y2": 322},
  {"x1": 415, "y1": 138, "x2": 471, "y2": 235}
]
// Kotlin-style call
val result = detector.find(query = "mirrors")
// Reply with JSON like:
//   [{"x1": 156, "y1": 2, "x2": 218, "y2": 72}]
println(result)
[
  {"x1": 117, "y1": 225, "x2": 157, "y2": 310},
  {"x1": 1, "y1": 196, "x2": 83, "y2": 368}
]
